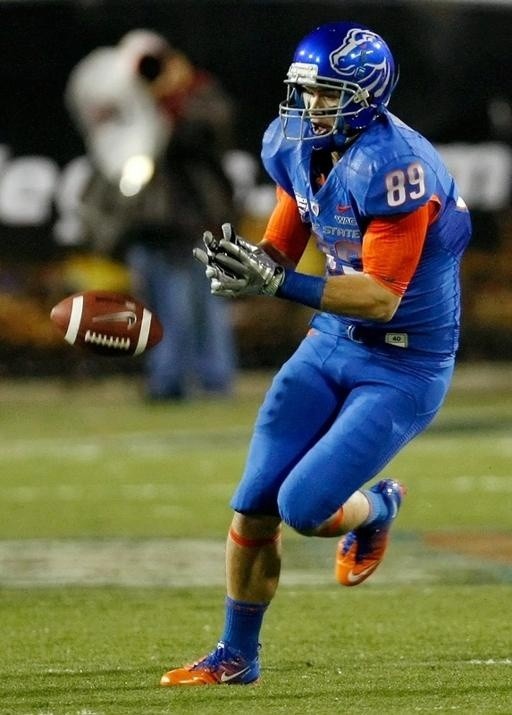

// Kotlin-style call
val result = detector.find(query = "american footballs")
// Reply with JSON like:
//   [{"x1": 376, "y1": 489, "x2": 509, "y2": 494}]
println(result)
[{"x1": 50, "y1": 290, "x2": 162, "y2": 357}]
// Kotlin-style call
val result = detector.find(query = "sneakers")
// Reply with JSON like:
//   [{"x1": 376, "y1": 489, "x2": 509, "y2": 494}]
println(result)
[
  {"x1": 335, "y1": 479, "x2": 406, "y2": 586},
  {"x1": 160, "y1": 643, "x2": 263, "y2": 687}
]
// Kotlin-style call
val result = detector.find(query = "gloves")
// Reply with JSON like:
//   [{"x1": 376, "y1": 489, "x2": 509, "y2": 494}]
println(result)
[{"x1": 194, "y1": 224, "x2": 285, "y2": 297}]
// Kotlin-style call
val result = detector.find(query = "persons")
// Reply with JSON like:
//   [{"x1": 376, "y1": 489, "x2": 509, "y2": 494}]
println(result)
[
  {"x1": 159, "y1": 21, "x2": 474, "y2": 687},
  {"x1": 66, "y1": 29, "x2": 239, "y2": 404}
]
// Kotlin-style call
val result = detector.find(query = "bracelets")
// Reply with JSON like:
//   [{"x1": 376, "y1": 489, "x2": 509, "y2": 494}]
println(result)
[{"x1": 274, "y1": 270, "x2": 327, "y2": 313}]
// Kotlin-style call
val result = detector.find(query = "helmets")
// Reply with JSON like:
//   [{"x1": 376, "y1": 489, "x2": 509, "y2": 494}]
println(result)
[{"x1": 278, "y1": 23, "x2": 399, "y2": 151}]
